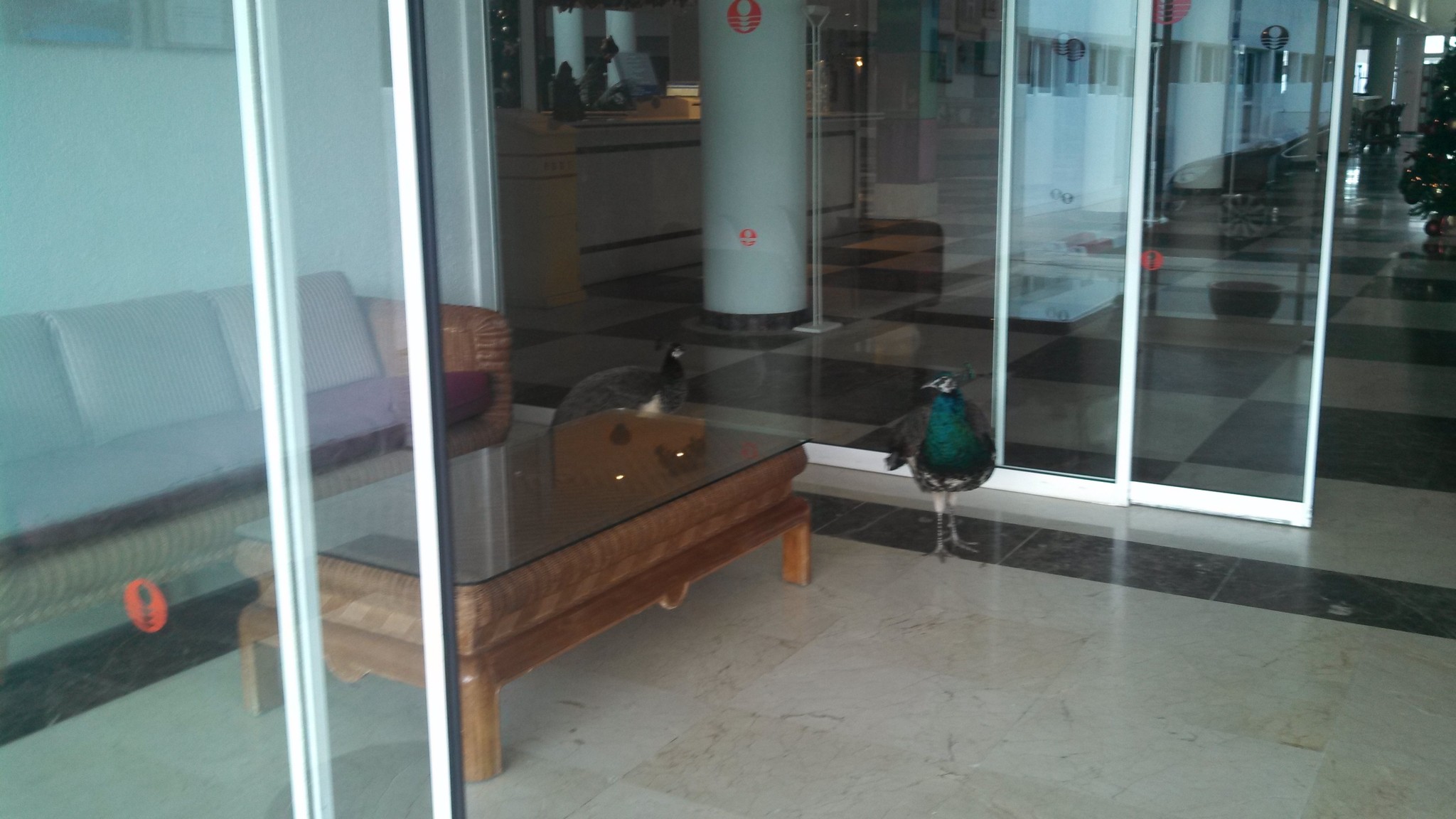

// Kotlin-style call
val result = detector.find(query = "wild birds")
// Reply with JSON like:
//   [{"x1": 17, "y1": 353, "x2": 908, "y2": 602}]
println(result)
[
  {"x1": 881, "y1": 373, "x2": 997, "y2": 564},
  {"x1": 548, "y1": 342, "x2": 687, "y2": 424}
]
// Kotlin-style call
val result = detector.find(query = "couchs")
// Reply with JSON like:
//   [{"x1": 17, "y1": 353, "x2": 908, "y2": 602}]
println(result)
[{"x1": 0, "y1": 270, "x2": 512, "y2": 636}]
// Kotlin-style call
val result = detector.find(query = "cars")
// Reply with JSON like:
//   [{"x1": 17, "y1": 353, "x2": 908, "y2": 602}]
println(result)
[{"x1": 1162, "y1": 122, "x2": 1434, "y2": 228}]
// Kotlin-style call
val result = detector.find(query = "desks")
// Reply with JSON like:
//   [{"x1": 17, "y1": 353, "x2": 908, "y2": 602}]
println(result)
[{"x1": 232, "y1": 408, "x2": 812, "y2": 783}]
